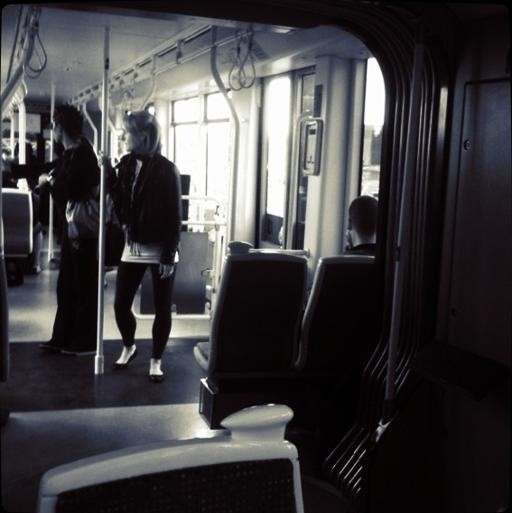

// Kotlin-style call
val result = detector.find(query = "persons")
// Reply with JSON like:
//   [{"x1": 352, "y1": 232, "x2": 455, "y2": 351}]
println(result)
[
  {"x1": 0, "y1": 101, "x2": 100, "y2": 356},
  {"x1": 343, "y1": 195, "x2": 380, "y2": 257},
  {"x1": 97, "y1": 109, "x2": 184, "y2": 385}
]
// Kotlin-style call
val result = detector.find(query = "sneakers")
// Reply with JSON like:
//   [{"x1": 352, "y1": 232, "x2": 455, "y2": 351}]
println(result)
[
  {"x1": 147, "y1": 358, "x2": 165, "y2": 382},
  {"x1": 114, "y1": 342, "x2": 138, "y2": 368}
]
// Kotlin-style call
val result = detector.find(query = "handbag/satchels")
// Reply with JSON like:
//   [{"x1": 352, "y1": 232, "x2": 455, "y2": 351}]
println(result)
[{"x1": 65, "y1": 191, "x2": 99, "y2": 244}]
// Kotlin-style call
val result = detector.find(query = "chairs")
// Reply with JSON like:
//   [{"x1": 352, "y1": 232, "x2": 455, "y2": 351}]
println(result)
[
  {"x1": 104, "y1": 219, "x2": 125, "y2": 288},
  {"x1": 298, "y1": 254, "x2": 383, "y2": 476},
  {"x1": 0, "y1": 187, "x2": 35, "y2": 287},
  {"x1": 193, "y1": 240, "x2": 307, "y2": 431},
  {"x1": 34, "y1": 404, "x2": 303, "y2": 513}
]
